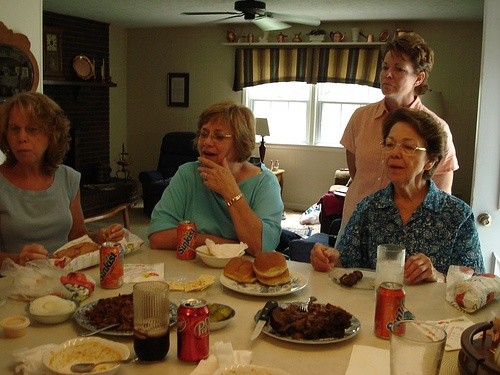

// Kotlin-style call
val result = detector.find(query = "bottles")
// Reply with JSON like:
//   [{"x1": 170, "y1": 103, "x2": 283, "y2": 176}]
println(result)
[
  {"x1": 270, "y1": 160, "x2": 274, "y2": 170},
  {"x1": 274, "y1": 161, "x2": 280, "y2": 168}
]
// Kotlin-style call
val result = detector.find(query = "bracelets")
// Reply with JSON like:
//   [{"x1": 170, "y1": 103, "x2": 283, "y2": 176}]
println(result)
[{"x1": 226, "y1": 193, "x2": 244, "y2": 207}]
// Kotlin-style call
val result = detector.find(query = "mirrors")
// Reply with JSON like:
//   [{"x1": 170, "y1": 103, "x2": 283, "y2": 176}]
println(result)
[
  {"x1": 72, "y1": 55, "x2": 94, "y2": 80},
  {"x1": 0, "y1": 21, "x2": 39, "y2": 104}
]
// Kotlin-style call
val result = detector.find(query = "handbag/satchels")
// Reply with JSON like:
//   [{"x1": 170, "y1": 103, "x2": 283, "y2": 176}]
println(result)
[{"x1": 299, "y1": 203, "x2": 321, "y2": 225}]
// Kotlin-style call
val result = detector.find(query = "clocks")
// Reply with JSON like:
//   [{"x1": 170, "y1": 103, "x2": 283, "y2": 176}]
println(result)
[{"x1": 44, "y1": 25, "x2": 66, "y2": 80}]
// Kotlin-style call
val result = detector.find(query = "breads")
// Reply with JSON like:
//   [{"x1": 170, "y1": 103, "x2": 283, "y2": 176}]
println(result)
[
  {"x1": 54, "y1": 242, "x2": 98, "y2": 258},
  {"x1": 223, "y1": 252, "x2": 291, "y2": 286}
]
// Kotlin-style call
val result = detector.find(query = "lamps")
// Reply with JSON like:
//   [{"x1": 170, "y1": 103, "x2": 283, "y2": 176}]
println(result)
[
  {"x1": 256, "y1": 118, "x2": 270, "y2": 163},
  {"x1": 419, "y1": 89, "x2": 448, "y2": 120}
]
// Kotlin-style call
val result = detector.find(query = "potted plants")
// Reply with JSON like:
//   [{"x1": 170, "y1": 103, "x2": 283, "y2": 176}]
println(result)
[{"x1": 306, "y1": 29, "x2": 325, "y2": 42}]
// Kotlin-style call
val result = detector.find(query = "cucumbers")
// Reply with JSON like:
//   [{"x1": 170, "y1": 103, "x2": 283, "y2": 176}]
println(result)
[{"x1": 208, "y1": 303, "x2": 234, "y2": 322}]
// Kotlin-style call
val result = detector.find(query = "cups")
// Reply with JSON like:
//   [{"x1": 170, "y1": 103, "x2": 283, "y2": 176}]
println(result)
[
  {"x1": 446, "y1": 265, "x2": 474, "y2": 302},
  {"x1": 374, "y1": 244, "x2": 406, "y2": 307},
  {"x1": 389, "y1": 320, "x2": 447, "y2": 375}
]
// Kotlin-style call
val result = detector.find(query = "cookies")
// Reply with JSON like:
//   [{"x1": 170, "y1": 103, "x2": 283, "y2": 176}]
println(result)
[{"x1": 169, "y1": 274, "x2": 215, "y2": 292}]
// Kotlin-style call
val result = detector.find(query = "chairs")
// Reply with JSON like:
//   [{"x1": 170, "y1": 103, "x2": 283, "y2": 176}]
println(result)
[{"x1": 282, "y1": 219, "x2": 342, "y2": 264}]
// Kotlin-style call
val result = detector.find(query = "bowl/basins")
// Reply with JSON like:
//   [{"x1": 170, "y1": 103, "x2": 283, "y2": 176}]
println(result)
[
  {"x1": 0, "y1": 315, "x2": 31, "y2": 337},
  {"x1": 196, "y1": 243, "x2": 245, "y2": 268},
  {"x1": 42, "y1": 337, "x2": 129, "y2": 375},
  {"x1": 25, "y1": 298, "x2": 77, "y2": 324}
]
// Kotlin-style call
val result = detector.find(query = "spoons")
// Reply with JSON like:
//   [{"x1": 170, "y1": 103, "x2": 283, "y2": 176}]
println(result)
[{"x1": 70, "y1": 359, "x2": 132, "y2": 372}]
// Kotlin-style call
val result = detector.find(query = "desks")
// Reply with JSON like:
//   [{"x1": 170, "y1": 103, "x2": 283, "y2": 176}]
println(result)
[
  {"x1": 80, "y1": 178, "x2": 139, "y2": 232},
  {"x1": 272, "y1": 169, "x2": 286, "y2": 220}
]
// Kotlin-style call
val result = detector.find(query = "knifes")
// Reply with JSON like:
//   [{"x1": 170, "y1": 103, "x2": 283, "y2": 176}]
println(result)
[{"x1": 249, "y1": 301, "x2": 277, "y2": 342}]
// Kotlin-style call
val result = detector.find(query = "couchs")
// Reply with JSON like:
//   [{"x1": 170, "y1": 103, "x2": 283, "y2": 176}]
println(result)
[
  {"x1": 138, "y1": 132, "x2": 201, "y2": 216},
  {"x1": 317, "y1": 170, "x2": 352, "y2": 234}
]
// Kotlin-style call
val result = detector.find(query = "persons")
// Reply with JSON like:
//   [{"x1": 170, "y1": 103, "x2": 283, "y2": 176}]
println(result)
[
  {"x1": 0, "y1": 91, "x2": 125, "y2": 273},
  {"x1": 332, "y1": 31, "x2": 460, "y2": 247},
  {"x1": 308, "y1": 107, "x2": 485, "y2": 275},
  {"x1": 146, "y1": 101, "x2": 284, "y2": 256}
]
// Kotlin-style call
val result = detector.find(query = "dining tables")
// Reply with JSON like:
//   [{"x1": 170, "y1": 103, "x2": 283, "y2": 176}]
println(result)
[{"x1": 0, "y1": 246, "x2": 499, "y2": 375}]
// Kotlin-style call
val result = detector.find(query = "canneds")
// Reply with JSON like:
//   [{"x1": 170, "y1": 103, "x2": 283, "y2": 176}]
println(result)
[
  {"x1": 177, "y1": 298, "x2": 210, "y2": 363},
  {"x1": 99, "y1": 242, "x2": 124, "y2": 289},
  {"x1": 176, "y1": 220, "x2": 196, "y2": 261},
  {"x1": 374, "y1": 281, "x2": 405, "y2": 340}
]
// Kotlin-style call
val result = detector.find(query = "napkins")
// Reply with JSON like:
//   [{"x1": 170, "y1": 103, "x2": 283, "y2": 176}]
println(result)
[
  {"x1": 12, "y1": 343, "x2": 58, "y2": 375},
  {"x1": 345, "y1": 344, "x2": 390, "y2": 375},
  {"x1": 190, "y1": 341, "x2": 253, "y2": 375}
]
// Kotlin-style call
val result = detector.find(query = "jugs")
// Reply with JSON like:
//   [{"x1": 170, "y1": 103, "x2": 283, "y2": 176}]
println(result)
[{"x1": 133, "y1": 281, "x2": 170, "y2": 363}]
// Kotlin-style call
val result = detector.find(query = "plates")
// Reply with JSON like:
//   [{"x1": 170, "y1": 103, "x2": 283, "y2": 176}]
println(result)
[
  {"x1": 328, "y1": 267, "x2": 375, "y2": 291},
  {"x1": 219, "y1": 270, "x2": 309, "y2": 297},
  {"x1": 206, "y1": 302, "x2": 238, "y2": 331},
  {"x1": 74, "y1": 299, "x2": 179, "y2": 336},
  {"x1": 254, "y1": 301, "x2": 361, "y2": 345}
]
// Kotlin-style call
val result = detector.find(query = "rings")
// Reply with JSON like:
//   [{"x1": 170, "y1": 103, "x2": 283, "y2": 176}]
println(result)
[
  {"x1": 25, "y1": 257, "x2": 30, "y2": 262},
  {"x1": 205, "y1": 172, "x2": 207, "y2": 181}
]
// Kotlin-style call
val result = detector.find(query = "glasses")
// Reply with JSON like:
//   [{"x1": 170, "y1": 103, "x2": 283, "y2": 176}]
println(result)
[
  {"x1": 196, "y1": 129, "x2": 234, "y2": 141},
  {"x1": 6, "y1": 123, "x2": 43, "y2": 136},
  {"x1": 380, "y1": 137, "x2": 427, "y2": 155}
]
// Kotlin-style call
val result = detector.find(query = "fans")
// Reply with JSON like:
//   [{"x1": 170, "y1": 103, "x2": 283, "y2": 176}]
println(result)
[{"x1": 182, "y1": 0, "x2": 320, "y2": 31}]
